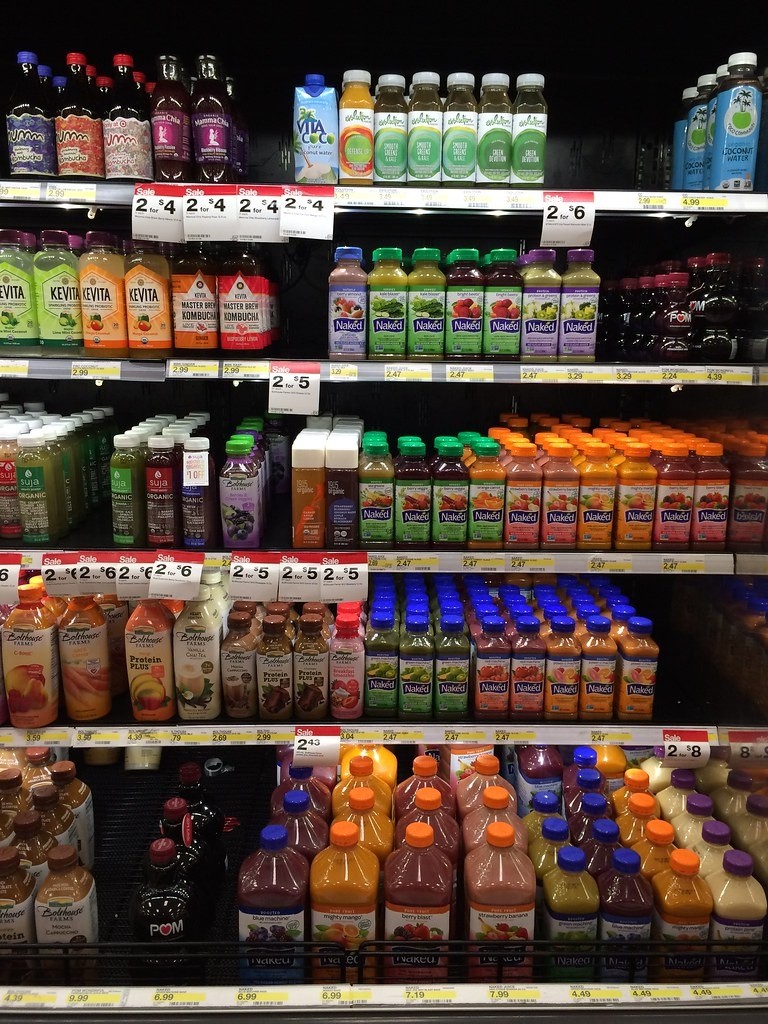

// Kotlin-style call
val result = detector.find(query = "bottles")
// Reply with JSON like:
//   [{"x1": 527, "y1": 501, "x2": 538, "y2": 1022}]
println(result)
[
  {"x1": 9, "y1": 48, "x2": 248, "y2": 185},
  {"x1": 338, "y1": 70, "x2": 549, "y2": 188},
  {"x1": 0, "y1": 572, "x2": 768, "y2": 724},
  {"x1": 0, "y1": 745, "x2": 768, "y2": 978},
  {"x1": 0, "y1": 387, "x2": 768, "y2": 548},
  {"x1": 672, "y1": 52, "x2": 768, "y2": 193},
  {"x1": 0, "y1": 224, "x2": 768, "y2": 363}
]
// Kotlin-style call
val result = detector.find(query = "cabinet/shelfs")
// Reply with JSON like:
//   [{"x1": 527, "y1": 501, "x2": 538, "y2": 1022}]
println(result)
[{"x1": 0, "y1": 180, "x2": 768, "y2": 1024}]
